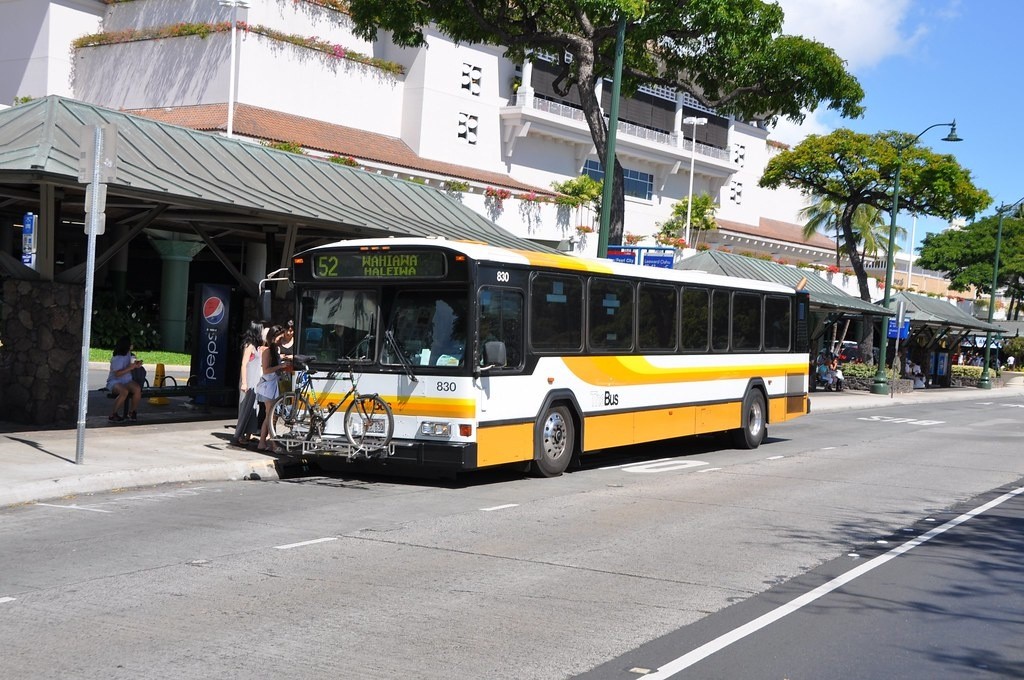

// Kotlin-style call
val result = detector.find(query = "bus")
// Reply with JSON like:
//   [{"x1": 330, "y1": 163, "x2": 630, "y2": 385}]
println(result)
[{"x1": 275, "y1": 235, "x2": 811, "y2": 479}]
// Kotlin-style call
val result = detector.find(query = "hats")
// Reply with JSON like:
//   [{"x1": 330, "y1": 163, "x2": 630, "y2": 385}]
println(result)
[
  {"x1": 819, "y1": 348, "x2": 827, "y2": 353},
  {"x1": 834, "y1": 353, "x2": 839, "y2": 356},
  {"x1": 907, "y1": 360, "x2": 911, "y2": 364}
]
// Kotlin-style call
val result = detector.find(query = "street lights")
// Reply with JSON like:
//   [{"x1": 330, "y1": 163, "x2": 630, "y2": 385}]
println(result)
[
  {"x1": 218, "y1": 0, "x2": 251, "y2": 139},
  {"x1": 870, "y1": 118, "x2": 964, "y2": 395},
  {"x1": 683, "y1": 116, "x2": 707, "y2": 246}
]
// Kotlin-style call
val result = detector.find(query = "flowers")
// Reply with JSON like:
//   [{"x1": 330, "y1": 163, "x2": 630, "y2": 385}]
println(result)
[
  {"x1": 827, "y1": 263, "x2": 856, "y2": 277},
  {"x1": 258, "y1": 138, "x2": 375, "y2": 173},
  {"x1": 695, "y1": 242, "x2": 711, "y2": 251},
  {"x1": 947, "y1": 295, "x2": 965, "y2": 304},
  {"x1": 73, "y1": 20, "x2": 408, "y2": 75},
  {"x1": 519, "y1": 190, "x2": 580, "y2": 209},
  {"x1": 765, "y1": 140, "x2": 790, "y2": 152},
  {"x1": 656, "y1": 235, "x2": 690, "y2": 249},
  {"x1": 623, "y1": 231, "x2": 646, "y2": 245},
  {"x1": 485, "y1": 185, "x2": 512, "y2": 211},
  {"x1": 718, "y1": 246, "x2": 790, "y2": 264},
  {"x1": 874, "y1": 273, "x2": 888, "y2": 290},
  {"x1": 575, "y1": 225, "x2": 595, "y2": 239}
]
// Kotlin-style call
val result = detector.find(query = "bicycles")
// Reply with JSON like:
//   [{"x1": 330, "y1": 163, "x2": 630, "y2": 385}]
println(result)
[{"x1": 267, "y1": 355, "x2": 394, "y2": 454}]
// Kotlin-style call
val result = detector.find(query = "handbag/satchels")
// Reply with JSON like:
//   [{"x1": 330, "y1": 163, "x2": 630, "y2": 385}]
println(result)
[{"x1": 131, "y1": 365, "x2": 147, "y2": 389}]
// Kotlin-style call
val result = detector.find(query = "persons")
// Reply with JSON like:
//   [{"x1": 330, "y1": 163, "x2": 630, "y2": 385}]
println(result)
[
  {"x1": 230, "y1": 319, "x2": 294, "y2": 455},
  {"x1": 952, "y1": 350, "x2": 1015, "y2": 376},
  {"x1": 809, "y1": 354, "x2": 844, "y2": 393},
  {"x1": 107, "y1": 335, "x2": 141, "y2": 423},
  {"x1": 449, "y1": 313, "x2": 499, "y2": 366},
  {"x1": 326, "y1": 319, "x2": 347, "y2": 362}
]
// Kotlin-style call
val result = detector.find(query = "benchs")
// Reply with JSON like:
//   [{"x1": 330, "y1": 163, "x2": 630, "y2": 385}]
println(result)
[
  {"x1": 102, "y1": 375, "x2": 224, "y2": 421},
  {"x1": 815, "y1": 378, "x2": 840, "y2": 391}
]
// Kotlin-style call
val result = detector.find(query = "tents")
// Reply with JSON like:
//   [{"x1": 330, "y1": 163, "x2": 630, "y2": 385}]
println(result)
[
  {"x1": 672, "y1": 249, "x2": 898, "y2": 344},
  {"x1": 872, "y1": 292, "x2": 1024, "y2": 350},
  {"x1": 0, "y1": 94, "x2": 569, "y2": 313}
]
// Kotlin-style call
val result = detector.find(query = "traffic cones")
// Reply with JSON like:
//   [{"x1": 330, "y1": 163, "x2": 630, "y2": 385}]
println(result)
[{"x1": 147, "y1": 364, "x2": 173, "y2": 404}]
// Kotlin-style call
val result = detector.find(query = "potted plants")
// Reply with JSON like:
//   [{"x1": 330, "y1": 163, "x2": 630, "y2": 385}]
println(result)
[{"x1": 1000, "y1": 336, "x2": 1024, "y2": 387}]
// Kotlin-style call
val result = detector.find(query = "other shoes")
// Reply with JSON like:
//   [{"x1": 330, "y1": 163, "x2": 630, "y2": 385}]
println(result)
[
  {"x1": 239, "y1": 437, "x2": 251, "y2": 444},
  {"x1": 127, "y1": 410, "x2": 137, "y2": 421},
  {"x1": 824, "y1": 386, "x2": 832, "y2": 392},
  {"x1": 109, "y1": 413, "x2": 124, "y2": 421},
  {"x1": 836, "y1": 387, "x2": 844, "y2": 391}
]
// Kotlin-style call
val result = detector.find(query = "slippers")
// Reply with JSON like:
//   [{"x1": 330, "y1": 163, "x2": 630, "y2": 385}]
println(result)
[
  {"x1": 257, "y1": 446, "x2": 272, "y2": 451},
  {"x1": 269, "y1": 447, "x2": 284, "y2": 454}
]
